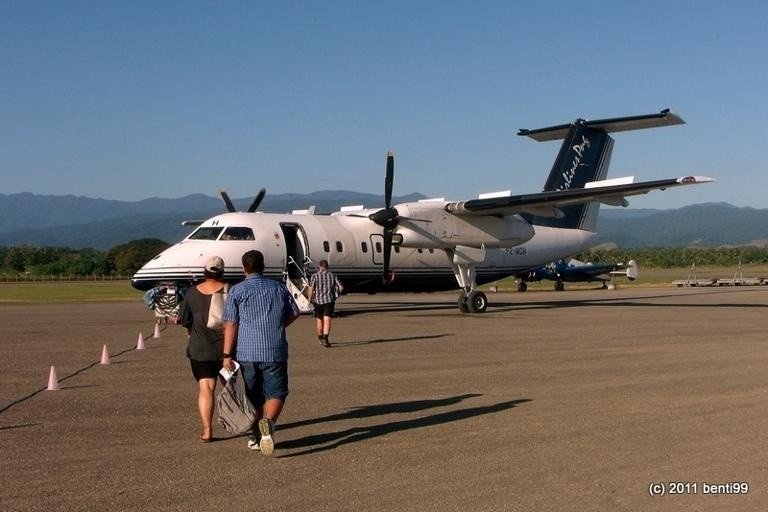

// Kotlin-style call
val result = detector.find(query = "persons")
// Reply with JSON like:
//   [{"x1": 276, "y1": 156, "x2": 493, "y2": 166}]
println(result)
[
  {"x1": 222, "y1": 233, "x2": 229, "y2": 241},
  {"x1": 178, "y1": 256, "x2": 231, "y2": 443},
  {"x1": 307, "y1": 259, "x2": 344, "y2": 347},
  {"x1": 221, "y1": 248, "x2": 300, "y2": 457}
]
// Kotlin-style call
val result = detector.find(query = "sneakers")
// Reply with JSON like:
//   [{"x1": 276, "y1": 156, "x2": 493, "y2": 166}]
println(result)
[
  {"x1": 258, "y1": 418, "x2": 275, "y2": 457},
  {"x1": 248, "y1": 440, "x2": 261, "y2": 452},
  {"x1": 319, "y1": 338, "x2": 331, "y2": 347}
]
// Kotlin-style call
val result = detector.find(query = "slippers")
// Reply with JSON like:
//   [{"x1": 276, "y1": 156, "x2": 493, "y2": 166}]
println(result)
[{"x1": 199, "y1": 435, "x2": 213, "y2": 444}]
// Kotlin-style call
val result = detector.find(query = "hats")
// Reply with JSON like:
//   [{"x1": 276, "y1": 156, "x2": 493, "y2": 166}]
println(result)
[
  {"x1": 205, "y1": 255, "x2": 225, "y2": 274},
  {"x1": 319, "y1": 260, "x2": 329, "y2": 268}
]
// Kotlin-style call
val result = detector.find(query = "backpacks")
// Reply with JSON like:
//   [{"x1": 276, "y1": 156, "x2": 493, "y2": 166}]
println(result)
[{"x1": 217, "y1": 370, "x2": 258, "y2": 437}]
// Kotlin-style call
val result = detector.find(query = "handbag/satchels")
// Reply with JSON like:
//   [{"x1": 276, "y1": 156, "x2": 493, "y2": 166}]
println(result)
[
  {"x1": 334, "y1": 285, "x2": 339, "y2": 300},
  {"x1": 207, "y1": 292, "x2": 230, "y2": 336}
]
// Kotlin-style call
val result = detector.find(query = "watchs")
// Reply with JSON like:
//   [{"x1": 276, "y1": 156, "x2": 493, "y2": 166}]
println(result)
[{"x1": 221, "y1": 352, "x2": 231, "y2": 358}]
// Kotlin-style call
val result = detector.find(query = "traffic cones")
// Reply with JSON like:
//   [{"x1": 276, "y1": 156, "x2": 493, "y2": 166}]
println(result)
[
  {"x1": 46, "y1": 366, "x2": 60, "y2": 391},
  {"x1": 98, "y1": 344, "x2": 111, "y2": 365},
  {"x1": 135, "y1": 333, "x2": 146, "y2": 349},
  {"x1": 154, "y1": 323, "x2": 161, "y2": 339}
]
